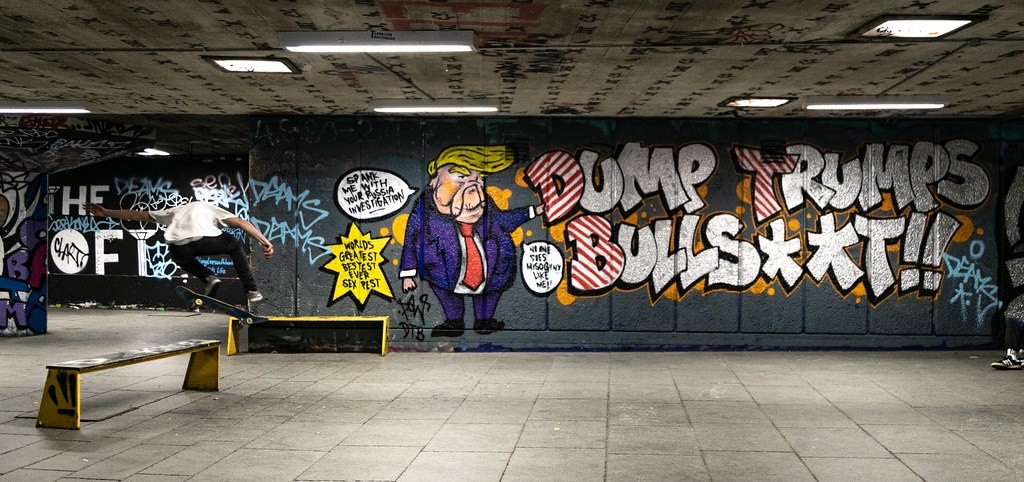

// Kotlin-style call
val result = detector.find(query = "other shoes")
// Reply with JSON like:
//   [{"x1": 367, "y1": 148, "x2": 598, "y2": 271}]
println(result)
[
  {"x1": 204, "y1": 275, "x2": 221, "y2": 296},
  {"x1": 249, "y1": 289, "x2": 263, "y2": 302}
]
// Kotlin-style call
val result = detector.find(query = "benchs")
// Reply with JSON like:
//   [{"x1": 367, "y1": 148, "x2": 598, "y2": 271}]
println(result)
[
  {"x1": 227, "y1": 316, "x2": 390, "y2": 356},
  {"x1": 36, "y1": 338, "x2": 221, "y2": 430}
]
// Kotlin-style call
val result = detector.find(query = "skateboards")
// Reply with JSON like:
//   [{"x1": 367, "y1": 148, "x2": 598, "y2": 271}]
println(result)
[{"x1": 175, "y1": 286, "x2": 268, "y2": 325}]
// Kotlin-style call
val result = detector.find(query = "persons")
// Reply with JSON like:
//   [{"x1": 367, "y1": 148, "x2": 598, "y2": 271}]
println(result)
[
  {"x1": 991, "y1": 316, "x2": 1024, "y2": 369},
  {"x1": 81, "y1": 200, "x2": 275, "y2": 301}
]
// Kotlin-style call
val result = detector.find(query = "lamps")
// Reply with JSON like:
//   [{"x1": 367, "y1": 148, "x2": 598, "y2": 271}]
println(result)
[
  {"x1": 275, "y1": 31, "x2": 480, "y2": 53},
  {"x1": 373, "y1": 99, "x2": 502, "y2": 113},
  {"x1": 0, "y1": 100, "x2": 91, "y2": 113},
  {"x1": 804, "y1": 95, "x2": 949, "y2": 110}
]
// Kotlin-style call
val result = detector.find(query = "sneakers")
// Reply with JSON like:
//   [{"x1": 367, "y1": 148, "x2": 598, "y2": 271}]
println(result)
[{"x1": 991, "y1": 348, "x2": 1024, "y2": 369}]
